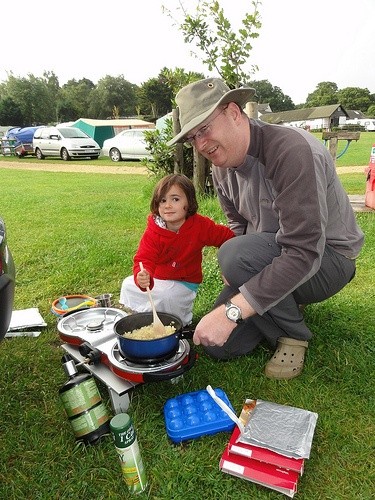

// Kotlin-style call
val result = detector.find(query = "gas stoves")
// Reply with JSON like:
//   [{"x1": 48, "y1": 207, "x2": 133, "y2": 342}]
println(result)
[{"x1": 58, "y1": 306, "x2": 197, "y2": 383}]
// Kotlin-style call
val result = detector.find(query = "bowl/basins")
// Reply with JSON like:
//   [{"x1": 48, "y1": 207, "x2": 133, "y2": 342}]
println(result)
[{"x1": 114, "y1": 312, "x2": 195, "y2": 362}]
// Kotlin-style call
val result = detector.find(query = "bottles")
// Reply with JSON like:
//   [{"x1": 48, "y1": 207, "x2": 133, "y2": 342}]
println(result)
[{"x1": 110, "y1": 413, "x2": 148, "y2": 494}]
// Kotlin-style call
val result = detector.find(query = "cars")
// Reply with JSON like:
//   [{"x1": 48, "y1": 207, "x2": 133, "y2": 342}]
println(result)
[{"x1": 102, "y1": 128, "x2": 169, "y2": 162}]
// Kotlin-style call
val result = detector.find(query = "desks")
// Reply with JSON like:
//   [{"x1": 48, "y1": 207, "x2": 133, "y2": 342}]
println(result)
[{"x1": 62, "y1": 342, "x2": 143, "y2": 416}]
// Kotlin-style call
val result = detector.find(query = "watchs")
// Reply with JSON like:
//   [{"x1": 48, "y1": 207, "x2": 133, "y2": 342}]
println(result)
[{"x1": 225, "y1": 300, "x2": 243, "y2": 325}]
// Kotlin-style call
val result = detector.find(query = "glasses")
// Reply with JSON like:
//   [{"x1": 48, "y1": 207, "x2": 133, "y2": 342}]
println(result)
[{"x1": 182, "y1": 107, "x2": 228, "y2": 148}]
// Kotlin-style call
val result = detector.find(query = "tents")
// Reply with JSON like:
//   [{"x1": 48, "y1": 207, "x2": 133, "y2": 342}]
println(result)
[{"x1": 71, "y1": 118, "x2": 155, "y2": 150}]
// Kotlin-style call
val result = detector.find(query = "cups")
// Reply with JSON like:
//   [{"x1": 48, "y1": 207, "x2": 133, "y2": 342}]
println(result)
[{"x1": 94, "y1": 293, "x2": 115, "y2": 308}]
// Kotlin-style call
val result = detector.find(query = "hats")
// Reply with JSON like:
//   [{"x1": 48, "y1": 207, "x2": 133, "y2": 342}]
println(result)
[{"x1": 165, "y1": 78, "x2": 256, "y2": 147}]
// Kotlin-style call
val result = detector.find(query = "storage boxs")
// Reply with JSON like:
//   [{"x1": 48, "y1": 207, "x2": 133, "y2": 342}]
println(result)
[{"x1": 220, "y1": 398, "x2": 305, "y2": 499}]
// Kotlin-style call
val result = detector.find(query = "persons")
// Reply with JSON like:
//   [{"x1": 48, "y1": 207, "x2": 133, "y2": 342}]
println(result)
[
  {"x1": 119, "y1": 173, "x2": 236, "y2": 332},
  {"x1": 168, "y1": 78, "x2": 364, "y2": 380}
]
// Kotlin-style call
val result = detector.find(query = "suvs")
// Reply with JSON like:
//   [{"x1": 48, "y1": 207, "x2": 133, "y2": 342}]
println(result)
[{"x1": 32, "y1": 127, "x2": 101, "y2": 161}]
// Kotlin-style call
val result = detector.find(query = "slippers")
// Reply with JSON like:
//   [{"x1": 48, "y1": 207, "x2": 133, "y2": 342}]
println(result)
[{"x1": 264, "y1": 337, "x2": 308, "y2": 378}]
